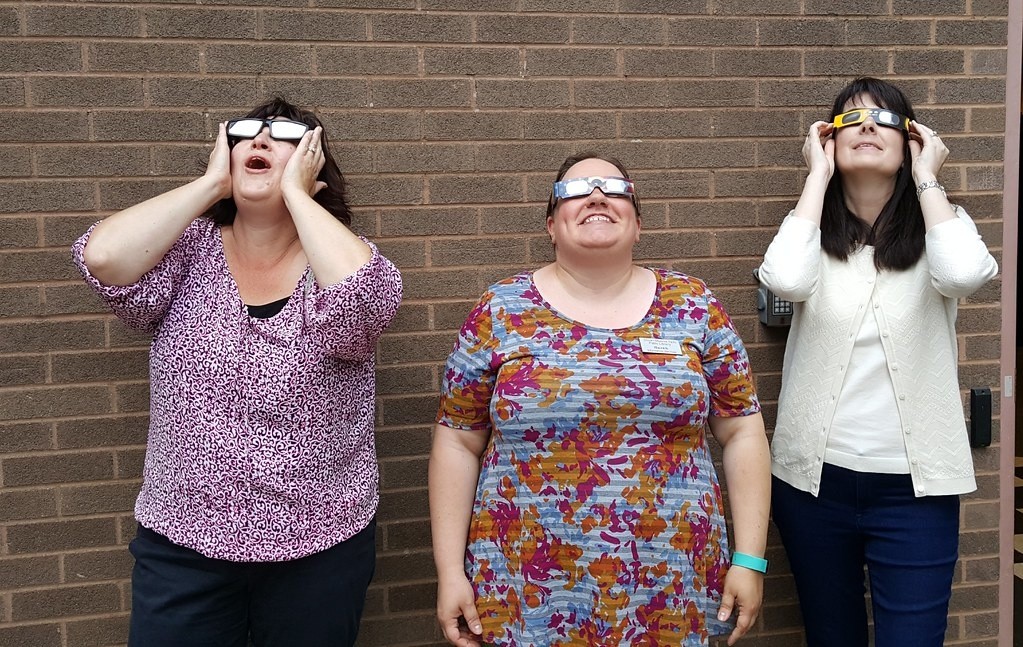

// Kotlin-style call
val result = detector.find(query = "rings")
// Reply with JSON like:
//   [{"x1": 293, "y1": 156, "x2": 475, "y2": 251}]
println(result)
[
  {"x1": 931, "y1": 131, "x2": 939, "y2": 137},
  {"x1": 307, "y1": 145, "x2": 317, "y2": 154}
]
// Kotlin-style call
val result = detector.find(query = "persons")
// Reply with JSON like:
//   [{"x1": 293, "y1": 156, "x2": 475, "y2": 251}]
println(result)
[
  {"x1": 759, "y1": 75, "x2": 999, "y2": 647},
  {"x1": 426, "y1": 152, "x2": 772, "y2": 647},
  {"x1": 70, "y1": 98, "x2": 403, "y2": 647}
]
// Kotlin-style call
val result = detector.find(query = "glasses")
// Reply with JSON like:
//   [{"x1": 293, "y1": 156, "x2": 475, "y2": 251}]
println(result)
[
  {"x1": 551, "y1": 176, "x2": 641, "y2": 215},
  {"x1": 831, "y1": 109, "x2": 910, "y2": 137},
  {"x1": 226, "y1": 117, "x2": 309, "y2": 147}
]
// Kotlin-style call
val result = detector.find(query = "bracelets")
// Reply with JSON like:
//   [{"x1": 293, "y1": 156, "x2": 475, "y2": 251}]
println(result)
[
  {"x1": 731, "y1": 552, "x2": 768, "y2": 573},
  {"x1": 916, "y1": 181, "x2": 945, "y2": 201}
]
